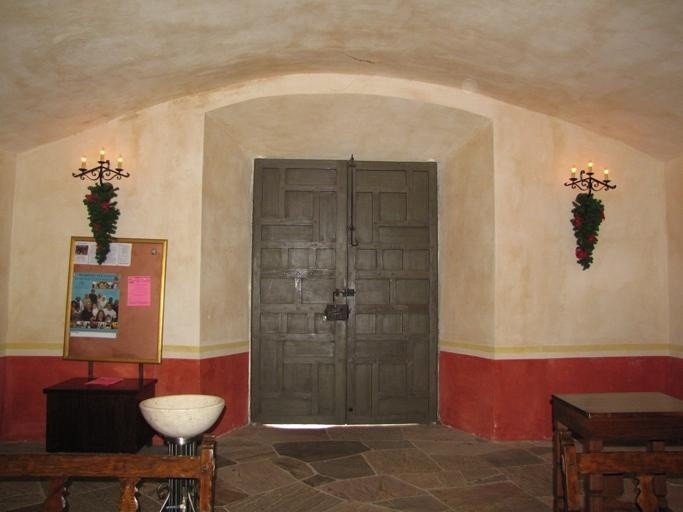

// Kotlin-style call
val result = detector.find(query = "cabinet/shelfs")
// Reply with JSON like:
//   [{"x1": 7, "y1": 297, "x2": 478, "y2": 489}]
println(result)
[{"x1": 43, "y1": 375, "x2": 158, "y2": 453}]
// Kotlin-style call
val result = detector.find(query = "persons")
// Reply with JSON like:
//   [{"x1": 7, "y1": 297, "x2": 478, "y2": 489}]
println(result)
[{"x1": 71, "y1": 288, "x2": 119, "y2": 328}]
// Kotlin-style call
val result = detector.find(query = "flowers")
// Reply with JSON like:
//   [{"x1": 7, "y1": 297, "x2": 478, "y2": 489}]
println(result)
[
  {"x1": 571, "y1": 191, "x2": 604, "y2": 271},
  {"x1": 83, "y1": 181, "x2": 118, "y2": 264}
]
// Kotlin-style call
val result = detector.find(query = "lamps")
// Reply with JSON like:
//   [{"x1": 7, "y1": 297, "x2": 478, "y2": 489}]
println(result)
[
  {"x1": 562, "y1": 161, "x2": 616, "y2": 197},
  {"x1": 72, "y1": 146, "x2": 129, "y2": 186}
]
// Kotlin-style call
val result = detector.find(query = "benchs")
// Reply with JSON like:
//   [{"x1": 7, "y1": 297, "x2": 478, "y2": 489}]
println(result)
[
  {"x1": 0, "y1": 437, "x2": 219, "y2": 511},
  {"x1": 561, "y1": 442, "x2": 683, "y2": 512}
]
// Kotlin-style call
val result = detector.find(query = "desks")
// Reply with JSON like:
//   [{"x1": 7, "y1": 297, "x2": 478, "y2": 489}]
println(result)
[{"x1": 551, "y1": 392, "x2": 683, "y2": 512}]
